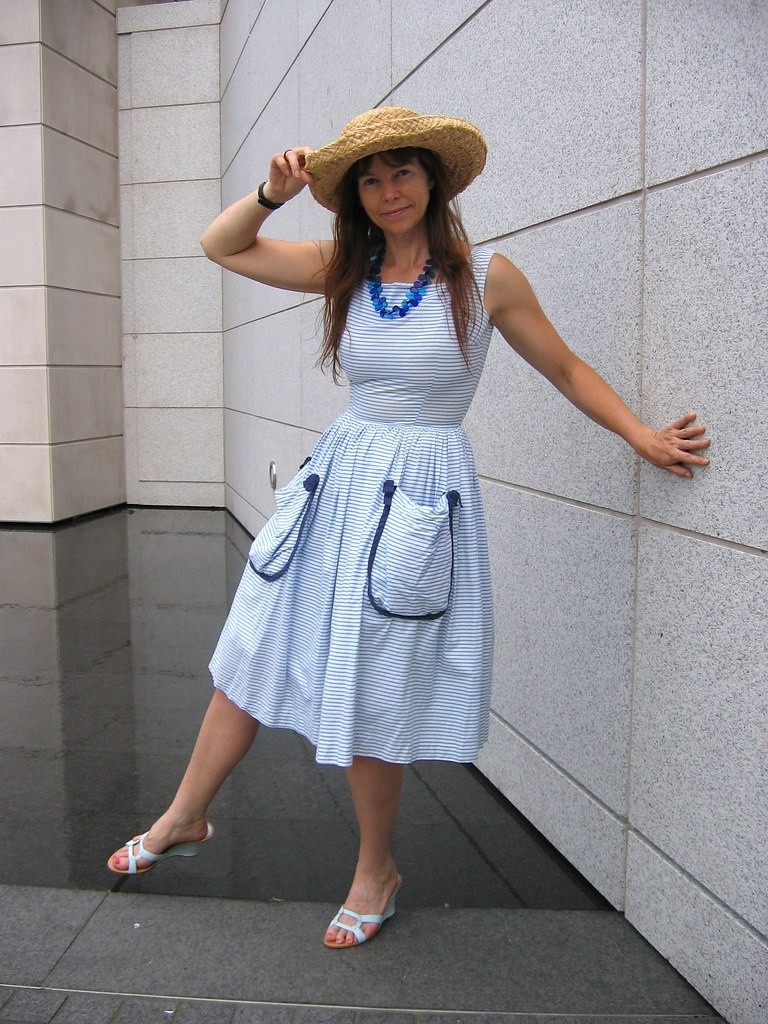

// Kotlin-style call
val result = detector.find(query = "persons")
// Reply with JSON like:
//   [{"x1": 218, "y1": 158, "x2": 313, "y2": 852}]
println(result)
[{"x1": 106, "y1": 107, "x2": 710, "y2": 948}]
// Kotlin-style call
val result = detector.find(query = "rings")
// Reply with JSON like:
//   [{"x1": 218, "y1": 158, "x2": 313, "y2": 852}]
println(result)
[{"x1": 283, "y1": 149, "x2": 292, "y2": 162}]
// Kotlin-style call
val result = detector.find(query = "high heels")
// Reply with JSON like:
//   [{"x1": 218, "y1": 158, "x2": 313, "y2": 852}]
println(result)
[
  {"x1": 322, "y1": 875, "x2": 404, "y2": 949},
  {"x1": 108, "y1": 820, "x2": 214, "y2": 874}
]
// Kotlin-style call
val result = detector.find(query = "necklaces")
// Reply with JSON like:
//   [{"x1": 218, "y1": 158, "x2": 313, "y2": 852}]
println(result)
[{"x1": 367, "y1": 253, "x2": 438, "y2": 319}]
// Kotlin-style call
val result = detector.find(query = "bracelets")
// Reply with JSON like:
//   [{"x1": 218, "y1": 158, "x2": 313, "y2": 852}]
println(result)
[{"x1": 258, "y1": 180, "x2": 285, "y2": 210}]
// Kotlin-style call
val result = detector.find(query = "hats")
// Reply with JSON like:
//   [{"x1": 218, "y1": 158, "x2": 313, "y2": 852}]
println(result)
[{"x1": 303, "y1": 107, "x2": 487, "y2": 215}]
